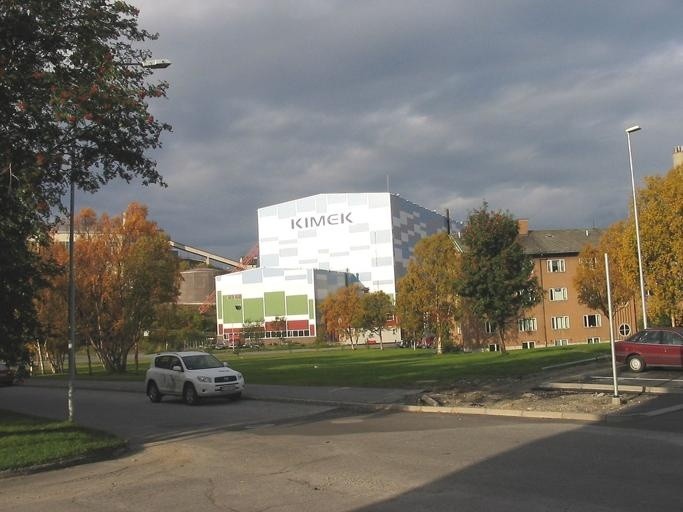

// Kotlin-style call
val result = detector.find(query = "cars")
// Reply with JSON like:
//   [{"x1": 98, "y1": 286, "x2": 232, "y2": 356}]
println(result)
[
  {"x1": 612, "y1": 325, "x2": 682, "y2": 374},
  {"x1": 0, "y1": 364, "x2": 14, "y2": 384},
  {"x1": 142, "y1": 348, "x2": 249, "y2": 404}
]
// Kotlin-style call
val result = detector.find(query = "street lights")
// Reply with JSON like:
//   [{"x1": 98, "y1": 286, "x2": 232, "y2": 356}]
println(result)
[
  {"x1": 624, "y1": 123, "x2": 649, "y2": 333},
  {"x1": 62, "y1": 54, "x2": 172, "y2": 425}
]
